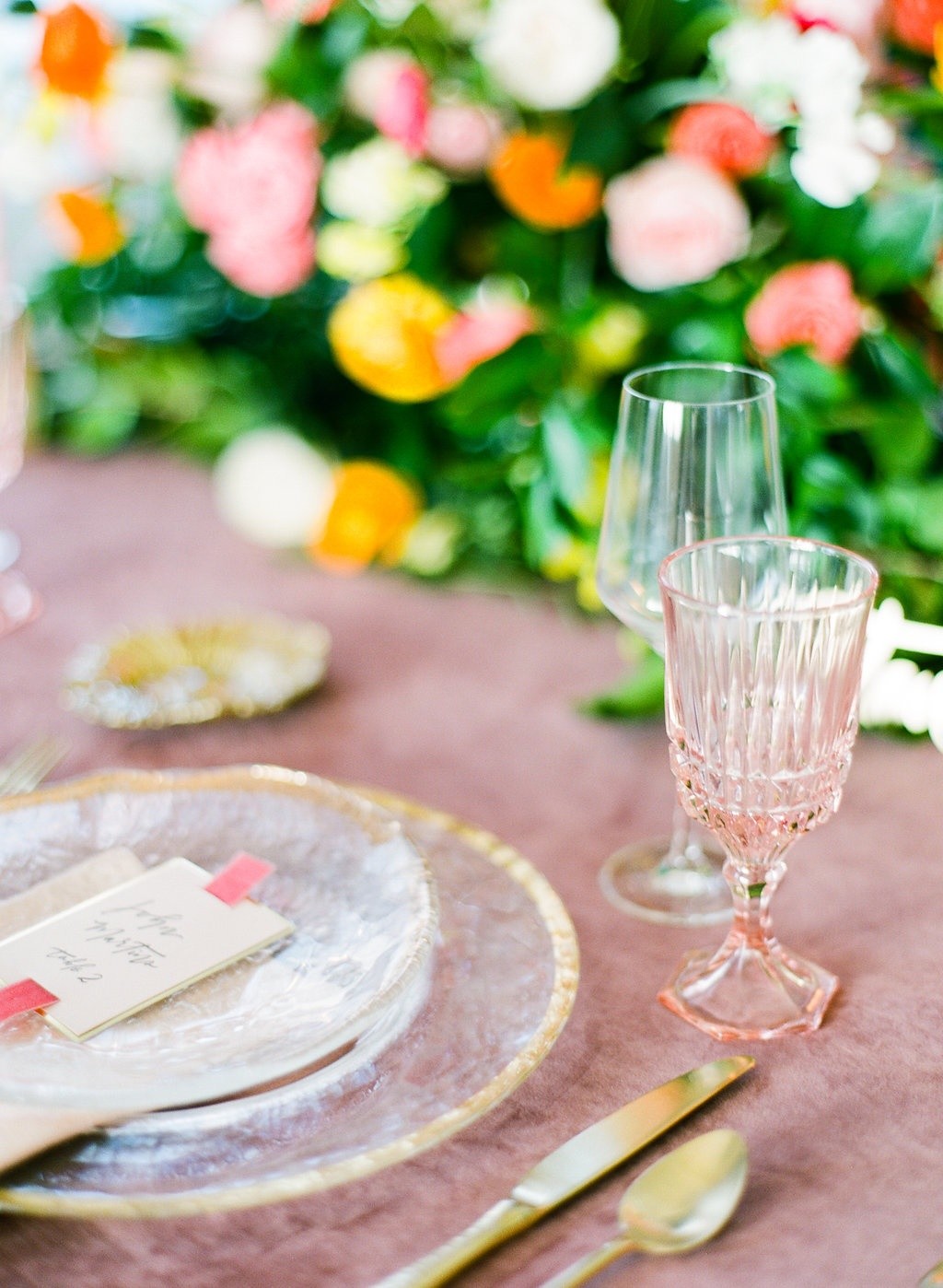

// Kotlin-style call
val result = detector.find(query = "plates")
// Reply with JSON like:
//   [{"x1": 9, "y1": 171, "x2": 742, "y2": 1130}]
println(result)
[{"x1": 1, "y1": 761, "x2": 582, "y2": 1223}]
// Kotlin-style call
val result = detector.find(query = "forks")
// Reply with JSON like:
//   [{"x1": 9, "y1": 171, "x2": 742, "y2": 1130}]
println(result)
[{"x1": 0, "y1": 741, "x2": 69, "y2": 798}]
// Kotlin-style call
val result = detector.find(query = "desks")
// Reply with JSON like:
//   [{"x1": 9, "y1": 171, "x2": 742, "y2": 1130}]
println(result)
[{"x1": 0, "y1": 440, "x2": 943, "y2": 1288}]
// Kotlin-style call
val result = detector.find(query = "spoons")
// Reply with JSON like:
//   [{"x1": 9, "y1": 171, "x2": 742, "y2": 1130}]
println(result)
[{"x1": 543, "y1": 1127, "x2": 749, "y2": 1288}]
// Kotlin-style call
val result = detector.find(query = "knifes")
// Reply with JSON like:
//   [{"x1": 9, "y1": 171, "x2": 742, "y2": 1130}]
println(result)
[{"x1": 364, "y1": 1054, "x2": 759, "y2": 1288}]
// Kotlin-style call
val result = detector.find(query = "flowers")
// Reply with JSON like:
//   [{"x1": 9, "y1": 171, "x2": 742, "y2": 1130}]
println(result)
[{"x1": 0, "y1": 0, "x2": 943, "y2": 754}]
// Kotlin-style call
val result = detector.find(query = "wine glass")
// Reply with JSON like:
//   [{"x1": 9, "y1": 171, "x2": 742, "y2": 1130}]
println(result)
[
  {"x1": 593, "y1": 362, "x2": 790, "y2": 927},
  {"x1": 658, "y1": 533, "x2": 879, "y2": 1040}
]
